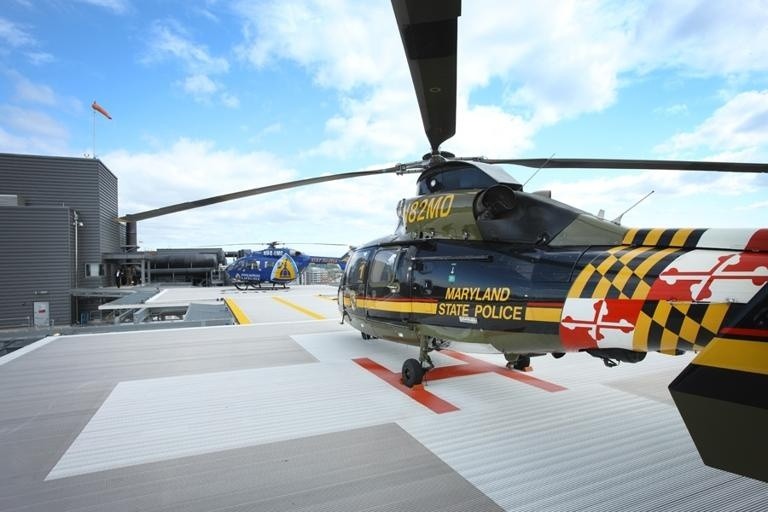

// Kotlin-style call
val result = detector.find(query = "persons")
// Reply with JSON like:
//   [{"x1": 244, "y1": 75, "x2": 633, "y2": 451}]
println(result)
[{"x1": 115, "y1": 267, "x2": 137, "y2": 288}]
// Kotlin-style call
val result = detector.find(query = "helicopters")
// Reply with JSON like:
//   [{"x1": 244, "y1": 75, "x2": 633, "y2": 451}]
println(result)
[
  {"x1": 191, "y1": 241, "x2": 347, "y2": 290},
  {"x1": 113, "y1": 0, "x2": 768, "y2": 482}
]
